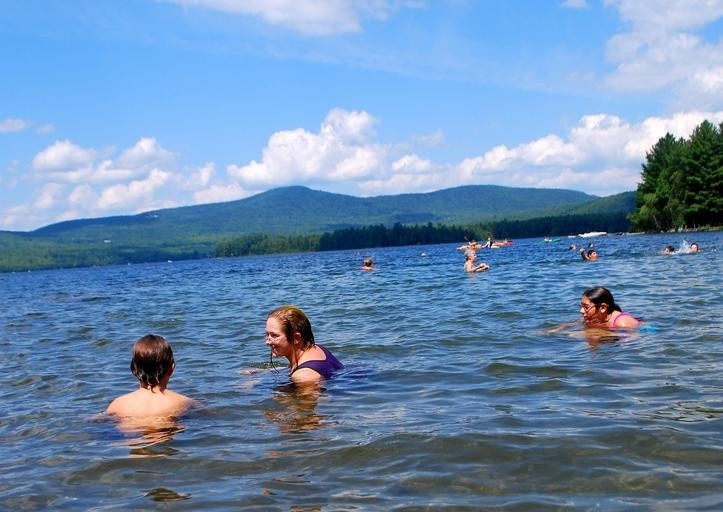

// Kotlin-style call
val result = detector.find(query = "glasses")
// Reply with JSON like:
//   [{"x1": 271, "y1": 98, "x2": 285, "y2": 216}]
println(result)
[{"x1": 579, "y1": 304, "x2": 596, "y2": 310}]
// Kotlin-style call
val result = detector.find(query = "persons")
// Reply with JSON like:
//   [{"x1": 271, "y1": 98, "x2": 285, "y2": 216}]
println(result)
[
  {"x1": 361, "y1": 259, "x2": 376, "y2": 270},
  {"x1": 663, "y1": 243, "x2": 699, "y2": 254},
  {"x1": 571, "y1": 244, "x2": 594, "y2": 250},
  {"x1": 457, "y1": 237, "x2": 509, "y2": 250},
  {"x1": 464, "y1": 250, "x2": 489, "y2": 273},
  {"x1": 239, "y1": 307, "x2": 343, "y2": 389},
  {"x1": 579, "y1": 288, "x2": 639, "y2": 330},
  {"x1": 106, "y1": 335, "x2": 193, "y2": 427},
  {"x1": 580, "y1": 249, "x2": 597, "y2": 261}
]
